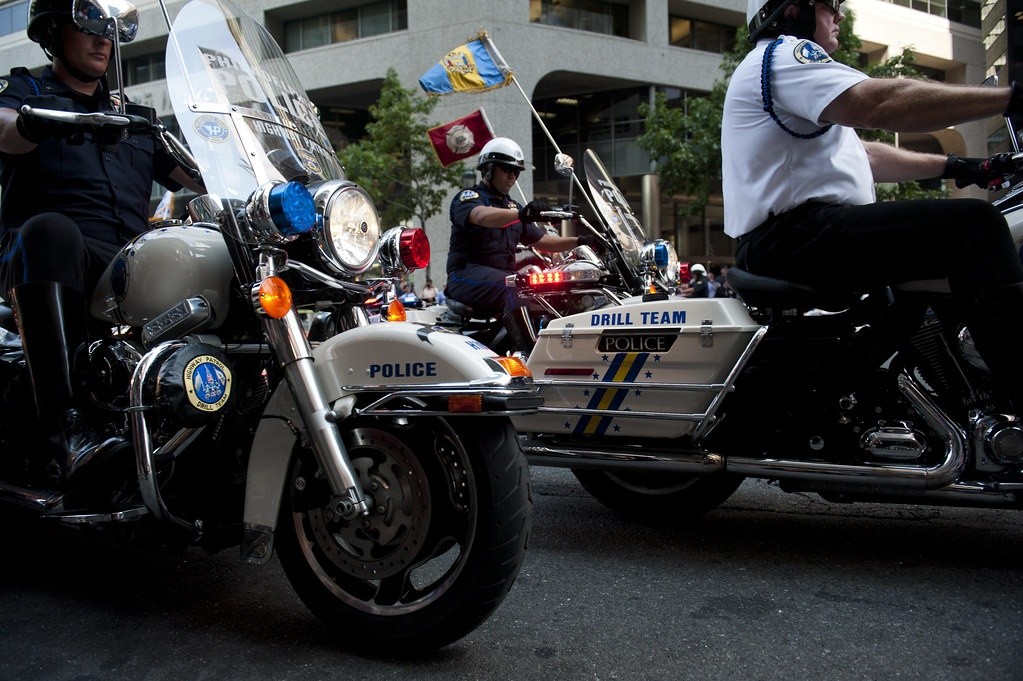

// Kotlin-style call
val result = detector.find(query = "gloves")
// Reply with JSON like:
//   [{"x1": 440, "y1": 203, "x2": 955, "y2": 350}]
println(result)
[
  {"x1": 16, "y1": 95, "x2": 88, "y2": 146},
  {"x1": 518, "y1": 200, "x2": 553, "y2": 224},
  {"x1": 577, "y1": 235, "x2": 605, "y2": 254},
  {"x1": 937, "y1": 152, "x2": 1018, "y2": 190}
]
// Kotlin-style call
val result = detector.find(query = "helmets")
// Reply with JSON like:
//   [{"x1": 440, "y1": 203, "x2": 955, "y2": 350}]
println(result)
[
  {"x1": 26, "y1": 0, "x2": 114, "y2": 62},
  {"x1": 747, "y1": 0, "x2": 845, "y2": 43},
  {"x1": 691, "y1": 264, "x2": 705, "y2": 272},
  {"x1": 476, "y1": 137, "x2": 525, "y2": 170}
]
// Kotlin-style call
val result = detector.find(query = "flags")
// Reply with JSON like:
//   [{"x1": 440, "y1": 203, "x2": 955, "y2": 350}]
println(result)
[
  {"x1": 419, "y1": 33, "x2": 513, "y2": 95},
  {"x1": 426, "y1": 109, "x2": 494, "y2": 168}
]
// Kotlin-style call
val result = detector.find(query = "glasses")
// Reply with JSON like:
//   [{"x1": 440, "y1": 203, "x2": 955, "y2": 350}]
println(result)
[
  {"x1": 816, "y1": 0, "x2": 839, "y2": 12},
  {"x1": 497, "y1": 163, "x2": 520, "y2": 176}
]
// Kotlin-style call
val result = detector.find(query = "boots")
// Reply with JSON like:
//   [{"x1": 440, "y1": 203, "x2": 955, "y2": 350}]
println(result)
[
  {"x1": 975, "y1": 281, "x2": 1023, "y2": 418},
  {"x1": 8, "y1": 282, "x2": 136, "y2": 493},
  {"x1": 502, "y1": 307, "x2": 537, "y2": 357}
]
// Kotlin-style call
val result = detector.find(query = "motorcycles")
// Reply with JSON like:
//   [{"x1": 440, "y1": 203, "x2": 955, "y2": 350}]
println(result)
[
  {"x1": 366, "y1": 145, "x2": 682, "y2": 367},
  {"x1": 0, "y1": 0, "x2": 544, "y2": 654},
  {"x1": 509, "y1": 60, "x2": 1023, "y2": 516}
]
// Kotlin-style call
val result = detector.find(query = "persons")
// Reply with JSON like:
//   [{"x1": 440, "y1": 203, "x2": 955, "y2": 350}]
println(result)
[
  {"x1": 721, "y1": 0, "x2": 1023, "y2": 410},
  {"x1": 399, "y1": 279, "x2": 447, "y2": 307},
  {"x1": 0, "y1": 0, "x2": 209, "y2": 510},
  {"x1": 677, "y1": 264, "x2": 736, "y2": 298},
  {"x1": 444, "y1": 137, "x2": 607, "y2": 358}
]
